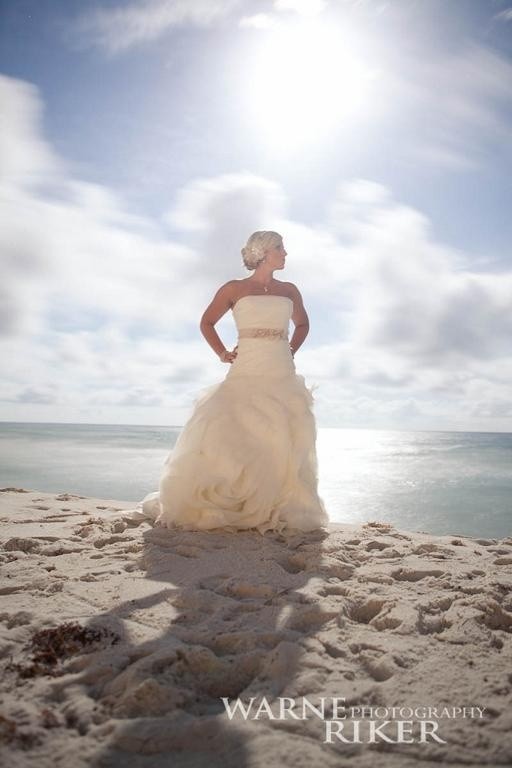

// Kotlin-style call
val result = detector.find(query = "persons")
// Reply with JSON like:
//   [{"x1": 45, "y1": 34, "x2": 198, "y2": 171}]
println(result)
[{"x1": 143, "y1": 231, "x2": 331, "y2": 535}]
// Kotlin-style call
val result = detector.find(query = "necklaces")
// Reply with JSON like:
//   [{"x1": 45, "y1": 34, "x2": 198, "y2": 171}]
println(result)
[{"x1": 251, "y1": 275, "x2": 274, "y2": 293}]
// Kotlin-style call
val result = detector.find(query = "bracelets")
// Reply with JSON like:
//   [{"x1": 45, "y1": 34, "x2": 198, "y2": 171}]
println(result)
[{"x1": 219, "y1": 350, "x2": 230, "y2": 362}]
